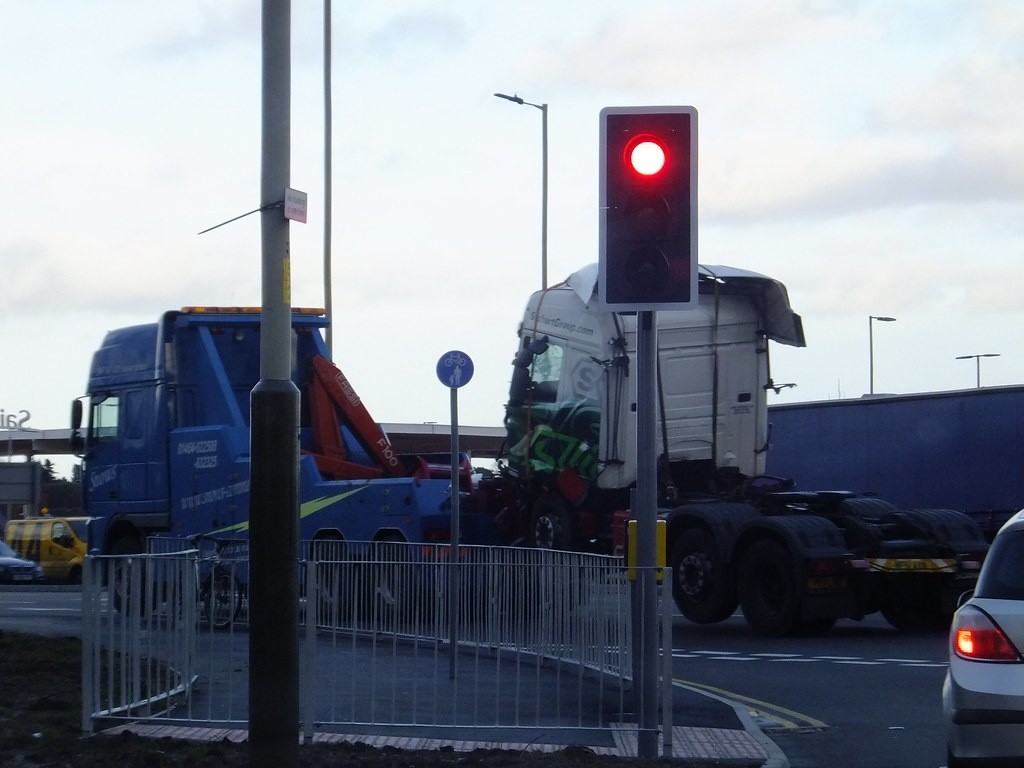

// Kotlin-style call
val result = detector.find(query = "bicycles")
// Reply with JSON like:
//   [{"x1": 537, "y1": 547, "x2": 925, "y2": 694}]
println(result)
[{"x1": 172, "y1": 534, "x2": 246, "y2": 630}]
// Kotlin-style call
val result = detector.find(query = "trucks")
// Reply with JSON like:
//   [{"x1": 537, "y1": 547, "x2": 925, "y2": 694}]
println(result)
[{"x1": 2, "y1": 517, "x2": 92, "y2": 586}]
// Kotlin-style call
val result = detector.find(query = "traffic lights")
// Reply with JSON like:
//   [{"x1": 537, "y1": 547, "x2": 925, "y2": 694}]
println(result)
[{"x1": 597, "y1": 105, "x2": 700, "y2": 312}]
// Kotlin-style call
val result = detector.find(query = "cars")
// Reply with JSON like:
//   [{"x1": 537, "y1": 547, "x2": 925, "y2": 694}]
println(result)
[
  {"x1": 0, "y1": 540, "x2": 45, "y2": 588},
  {"x1": 941, "y1": 509, "x2": 1024, "y2": 767}
]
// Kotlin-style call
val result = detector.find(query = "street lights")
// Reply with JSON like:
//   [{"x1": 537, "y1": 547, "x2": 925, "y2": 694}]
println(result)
[
  {"x1": 954, "y1": 353, "x2": 1001, "y2": 389},
  {"x1": 866, "y1": 313, "x2": 897, "y2": 396}
]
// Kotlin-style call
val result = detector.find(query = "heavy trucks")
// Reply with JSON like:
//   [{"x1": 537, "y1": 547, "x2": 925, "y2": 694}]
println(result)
[
  {"x1": 67, "y1": 305, "x2": 504, "y2": 631},
  {"x1": 503, "y1": 258, "x2": 985, "y2": 636}
]
